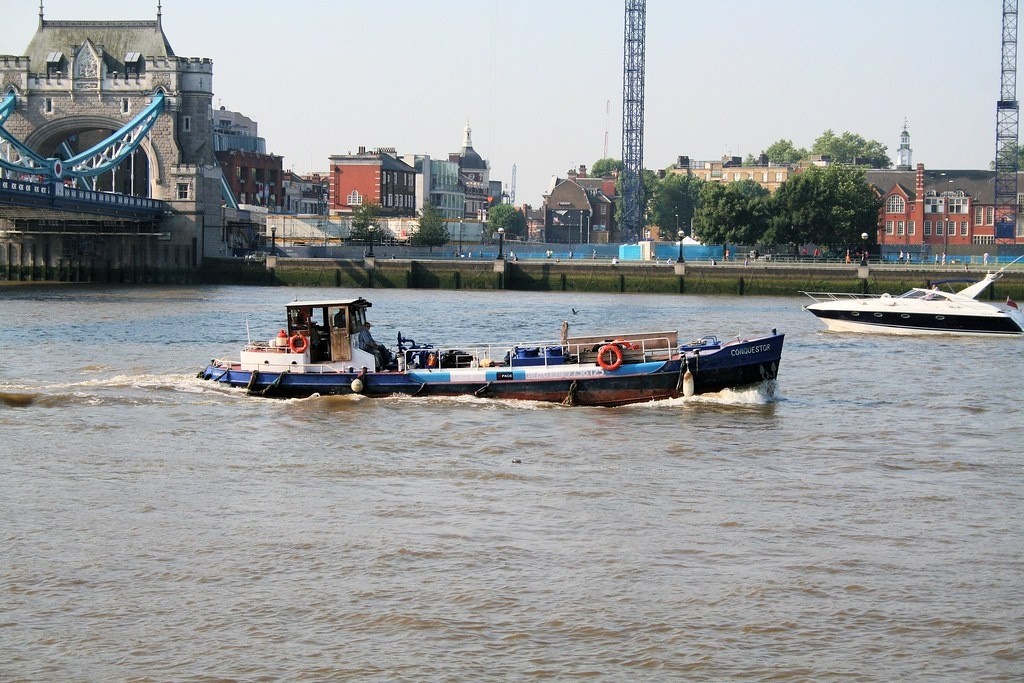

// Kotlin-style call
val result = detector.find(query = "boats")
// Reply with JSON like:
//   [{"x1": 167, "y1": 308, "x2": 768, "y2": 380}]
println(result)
[
  {"x1": 195, "y1": 298, "x2": 786, "y2": 408},
  {"x1": 797, "y1": 254, "x2": 1024, "y2": 337}
]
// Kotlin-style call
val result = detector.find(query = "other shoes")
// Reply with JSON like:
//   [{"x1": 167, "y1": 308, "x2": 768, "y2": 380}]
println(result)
[
  {"x1": 380, "y1": 369, "x2": 390, "y2": 372},
  {"x1": 385, "y1": 362, "x2": 393, "y2": 366}
]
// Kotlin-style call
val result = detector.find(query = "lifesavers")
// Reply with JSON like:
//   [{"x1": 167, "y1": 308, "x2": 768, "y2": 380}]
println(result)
[
  {"x1": 290, "y1": 334, "x2": 308, "y2": 353},
  {"x1": 597, "y1": 345, "x2": 622, "y2": 371},
  {"x1": 880, "y1": 292, "x2": 892, "y2": 302},
  {"x1": 610, "y1": 338, "x2": 632, "y2": 350}
]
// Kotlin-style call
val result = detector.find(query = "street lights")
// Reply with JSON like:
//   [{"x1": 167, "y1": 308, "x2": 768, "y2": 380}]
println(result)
[
  {"x1": 860, "y1": 233, "x2": 868, "y2": 266},
  {"x1": 677, "y1": 231, "x2": 685, "y2": 263},
  {"x1": 269, "y1": 225, "x2": 279, "y2": 256},
  {"x1": 496, "y1": 228, "x2": 505, "y2": 260},
  {"x1": 366, "y1": 225, "x2": 375, "y2": 257}
]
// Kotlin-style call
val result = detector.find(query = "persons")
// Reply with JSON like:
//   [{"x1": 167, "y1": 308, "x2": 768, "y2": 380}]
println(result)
[
  {"x1": 983, "y1": 250, "x2": 989, "y2": 266},
  {"x1": 592, "y1": 248, "x2": 597, "y2": 263},
  {"x1": 359, "y1": 322, "x2": 390, "y2": 373},
  {"x1": 667, "y1": 258, "x2": 672, "y2": 264},
  {"x1": 710, "y1": 258, "x2": 716, "y2": 266},
  {"x1": 846, "y1": 249, "x2": 872, "y2": 267},
  {"x1": 725, "y1": 249, "x2": 730, "y2": 261},
  {"x1": 569, "y1": 249, "x2": 573, "y2": 259},
  {"x1": 391, "y1": 253, "x2": 396, "y2": 260},
  {"x1": 612, "y1": 257, "x2": 619, "y2": 264},
  {"x1": 803, "y1": 248, "x2": 820, "y2": 255},
  {"x1": 964, "y1": 264, "x2": 968, "y2": 269},
  {"x1": 899, "y1": 251, "x2": 946, "y2": 268},
  {"x1": 455, "y1": 250, "x2": 483, "y2": 258},
  {"x1": 556, "y1": 259, "x2": 560, "y2": 262},
  {"x1": 334, "y1": 308, "x2": 346, "y2": 328},
  {"x1": 504, "y1": 249, "x2": 518, "y2": 262},
  {"x1": 546, "y1": 249, "x2": 551, "y2": 258},
  {"x1": 744, "y1": 250, "x2": 759, "y2": 267},
  {"x1": 650, "y1": 246, "x2": 657, "y2": 260}
]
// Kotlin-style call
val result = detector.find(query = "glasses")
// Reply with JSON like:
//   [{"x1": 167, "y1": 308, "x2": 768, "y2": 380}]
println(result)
[{"x1": 365, "y1": 326, "x2": 371, "y2": 327}]
